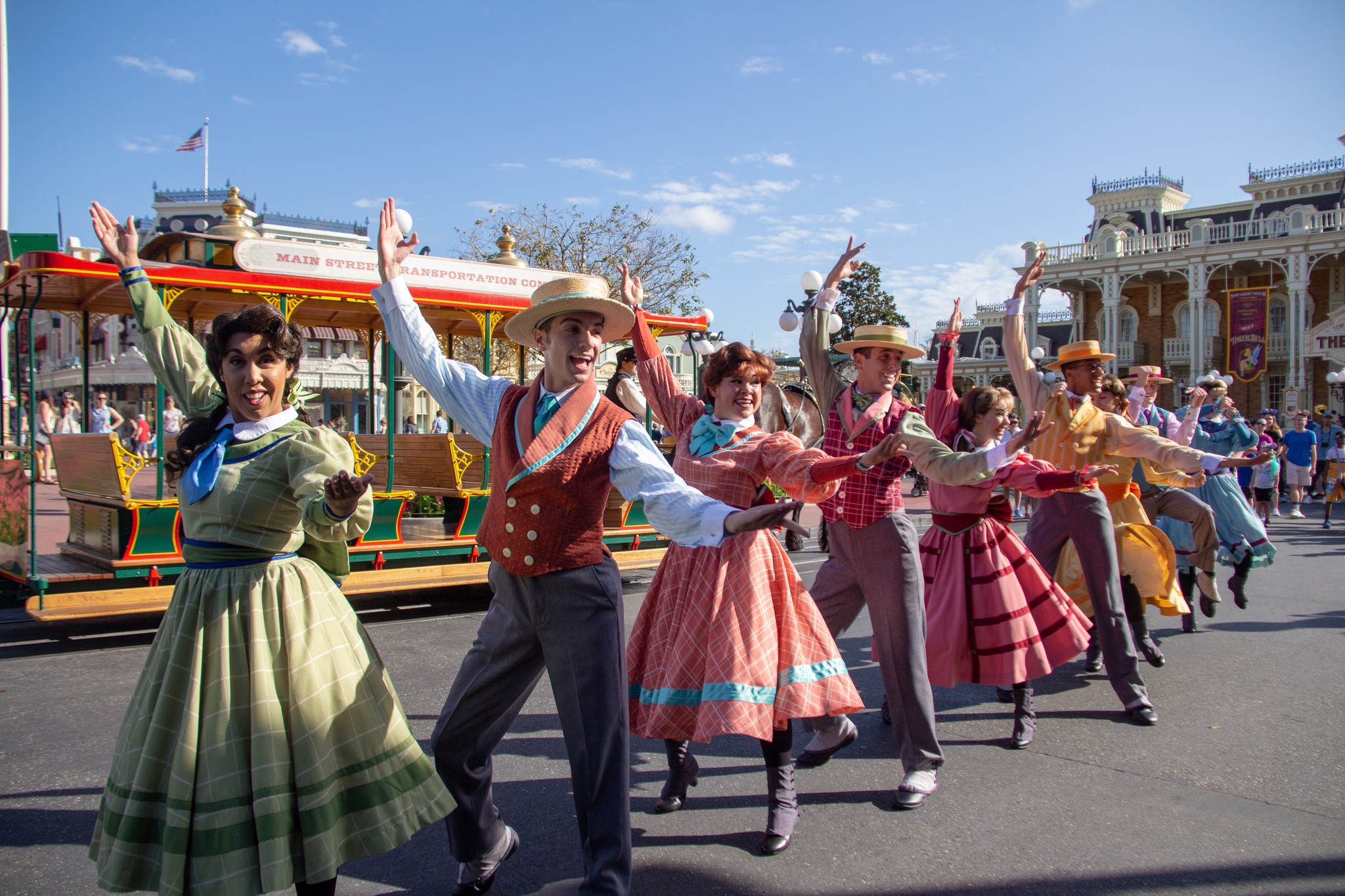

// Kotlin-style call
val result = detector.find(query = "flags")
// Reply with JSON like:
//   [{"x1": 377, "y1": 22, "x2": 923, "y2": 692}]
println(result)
[{"x1": 175, "y1": 129, "x2": 204, "y2": 152}]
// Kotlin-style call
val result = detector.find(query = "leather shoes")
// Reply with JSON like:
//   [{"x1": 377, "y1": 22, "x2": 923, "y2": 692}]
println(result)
[
  {"x1": 1012, "y1": 739, "x2": 1029, "y2": 750},
  {"x1": 451, "y1": 831, "x2": 519, "y2": 896},
  {"x1": 657, "y1": 578, "x2": 1246, "y2": 854}
]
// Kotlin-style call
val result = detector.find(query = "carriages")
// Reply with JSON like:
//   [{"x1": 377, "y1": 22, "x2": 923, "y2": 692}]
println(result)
[{"x1": 1, "y1": 183, "x2": 835, "y2": 627}]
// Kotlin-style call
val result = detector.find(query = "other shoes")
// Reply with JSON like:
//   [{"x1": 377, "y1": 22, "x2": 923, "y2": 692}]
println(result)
[
  {"x1": 136, "y1": 462, "x2": 153, "y2": 467},
  {"x1": 1260, "y1": 492, "x2": 1331, "y2": 529}
]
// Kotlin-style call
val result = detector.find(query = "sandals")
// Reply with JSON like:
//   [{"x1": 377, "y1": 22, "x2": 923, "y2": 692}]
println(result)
[{"x1": 37, "y1": 479, "x2": 59, "y2": 485}]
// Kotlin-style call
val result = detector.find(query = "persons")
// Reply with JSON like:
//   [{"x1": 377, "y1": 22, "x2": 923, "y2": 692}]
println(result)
[
  {"x1": 403, "y1": 410, "x2": 448, "y2": 434},
  {"x1": 7, "y1": 390, "x2": 184, "y2": 484},
  {"x1": 999, "y1": 413, "x2": 1037, "y2": 518},
  {"x1": 652, "y1": 422, "x2": 662, "y2": 444},
  {"x1": 604, "y1": 346, "x2": 664, "y2": 426},
  {"x1": 881, "y1": 295, "x2": 1120, "y2": 749},
  {"x1": 1124, "y1": 365, "x2": 1278, "y2": 633},
  {"x1": 997, "y1": 250, "x2": 1276, "y2": 724},
  {"x1": 461, "y1": 428, "x2": 469, "y2": 434},
  {"x1": 317, "y1": 417, "x2": 347, "y2": 432},
  {"x1": 613, "y1": 260, "x2": 917, "y2": 856},
  {"x1": 371, "y1": 196, "x2": 804, "y2": 895},
  {"x1": 1051, "y1": 374, "x2": 1206, "y2": 673},
  {"x1": 798, "y1": 235, "x2": 1056, "y2": 809},
  {"x1": 1235, "y1": 407, "x2": 1345, "y2": 528},
  {"x1": 377, "y1": 418, "x2": 387, "y2": 434},
  {"x1": 87, "y1": 201, "x2": 458, "y2": 896},
  {"x1": 660, "y1": 427, "x2": 665, "y2": 440}
]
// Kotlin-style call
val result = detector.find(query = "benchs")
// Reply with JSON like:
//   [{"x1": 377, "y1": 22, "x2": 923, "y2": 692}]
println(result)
[
  {"x1": 50, "y1": 428, "x2": 186, "y2": 571},
  {"x1": 357, "y1": 430, "x2": 497, "y2": 542},
  {"x1": 328, "y1": 425, "x2": 414, "y2": 548}
]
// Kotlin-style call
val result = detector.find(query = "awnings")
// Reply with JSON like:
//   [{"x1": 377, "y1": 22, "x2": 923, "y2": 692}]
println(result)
[{"x1": 184, "y1": 318, "x2": 358, "y2": 341}]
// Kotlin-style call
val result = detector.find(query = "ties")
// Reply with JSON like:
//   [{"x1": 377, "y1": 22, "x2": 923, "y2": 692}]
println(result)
[
  {"x1": 182, "y1": 428, "x2": 236, "y2": 506},
  {"x1": 1069, "y1": 396, "x2": 1081, "y2": 418},
  {"x1": 532, "y1": 396, "x2": 560, "y2": 437},
  {"x1": 1144, "y1": 410, "x2": 1151, "y2": 425}
]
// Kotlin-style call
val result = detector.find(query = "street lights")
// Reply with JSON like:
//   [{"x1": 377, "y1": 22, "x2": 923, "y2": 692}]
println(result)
[
  {"x1": 680, "y1": 307, "x2": 731, "y2": 397},
  {"x1": 1325, "y1": 367, "x2": 1345, "y2": 428}
]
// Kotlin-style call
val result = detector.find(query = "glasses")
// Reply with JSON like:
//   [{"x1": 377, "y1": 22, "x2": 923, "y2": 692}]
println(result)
[
  {"x1": 1256, "y1": 422, "x2": 1266, "y2": 427},
  {"x1": 97, "y1": 398, "x2": 107, "y2": 400},
  {"x1": 1071, "y1": 362, "x2": 1107, "y2": 373},
  {"x1": 630, "y1": 360, "x2": 638, "y2": 365}
]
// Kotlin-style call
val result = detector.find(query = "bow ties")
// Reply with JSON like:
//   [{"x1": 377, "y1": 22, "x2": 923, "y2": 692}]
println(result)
[{"x1": 851, "y1": 387, "x2": 874, "y2": 411}]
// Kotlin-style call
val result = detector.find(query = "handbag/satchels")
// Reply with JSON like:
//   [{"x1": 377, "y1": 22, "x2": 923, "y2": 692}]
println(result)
[{"x1": 34, "y1": 432, "x2": 49, "y2": 447}]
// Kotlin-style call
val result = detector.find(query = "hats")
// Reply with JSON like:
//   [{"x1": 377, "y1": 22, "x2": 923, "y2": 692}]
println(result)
[
  {"x1": 1323, "y1": 412, "x2": 1333, "y2": 418},
  {"x1": 832, "y1": 324, "x2": 927, "y2": 361},
  {"x1": 1046, "y1": 341, "x2": 1116, "y2": 370},
  {"x1": 136, "y1": 414, "x2": 146, "y2": 418},
  {"x1": 1120, "y1": 365, "x2": 1173, "y2": 384},
  {"x1": 615, "y1": 346, "x2": 636, "y2": 362},
  {"x1": 505, "y1": 275, "x2": 636, "y2": 348},
  {"x1": 7, "y1": 389, "x2": 74, "y2": 400}
]
